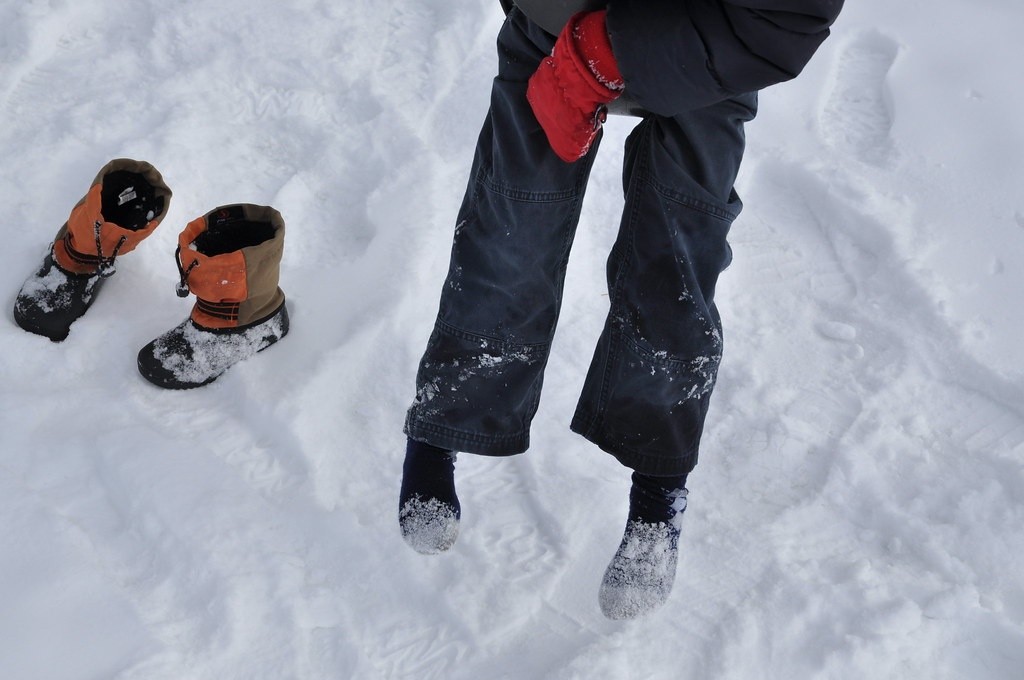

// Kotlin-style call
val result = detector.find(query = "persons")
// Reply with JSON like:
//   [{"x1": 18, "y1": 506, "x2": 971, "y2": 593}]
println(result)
[{"x1": 399, "y1": 0, "x2": 845, "y2": 619}]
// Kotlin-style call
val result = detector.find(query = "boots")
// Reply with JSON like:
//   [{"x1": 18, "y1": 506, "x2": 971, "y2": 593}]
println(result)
[
  {"x1": 137, "y1": 204, "x2": 289, "y2": 389},
  {"x1": 14, "y1": 158, "x2": 172, "y2": 337}
]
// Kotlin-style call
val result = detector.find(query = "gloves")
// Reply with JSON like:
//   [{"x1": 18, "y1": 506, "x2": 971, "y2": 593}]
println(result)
[{"x1": 525, "y1": 11, "x2": 622, "y2": 161}]
